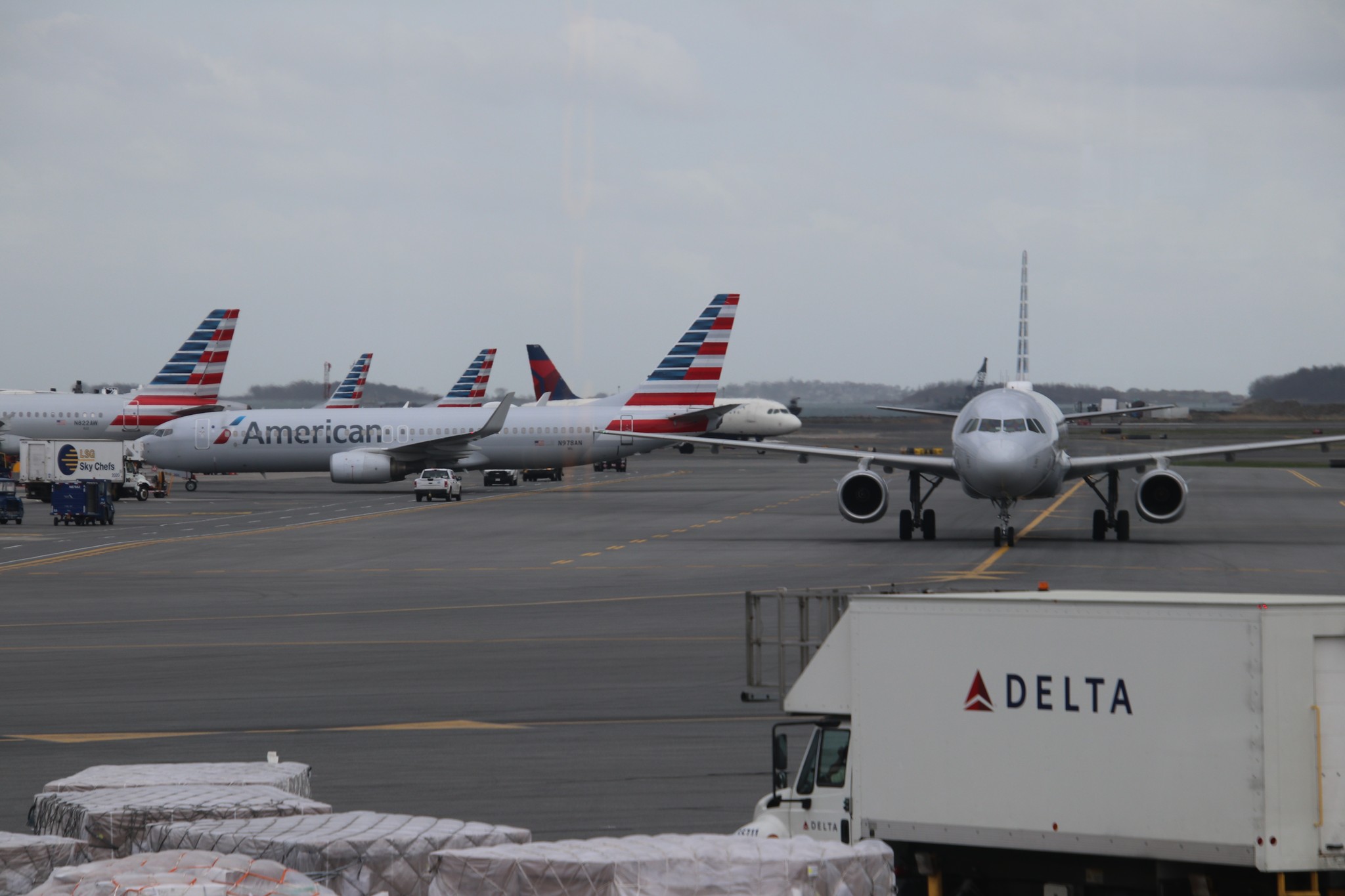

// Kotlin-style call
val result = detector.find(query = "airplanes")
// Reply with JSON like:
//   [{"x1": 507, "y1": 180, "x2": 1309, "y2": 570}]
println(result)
[
  {"x1": 599, "y1": 248, "x2": 1345, "y2": 550},
  {"x1": 0, "y1": 293, "x2": 803, "y2": 493}
]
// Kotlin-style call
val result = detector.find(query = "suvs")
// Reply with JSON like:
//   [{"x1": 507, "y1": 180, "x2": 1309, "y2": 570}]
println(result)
[{"x1": 483, "y1": 469, "x2": 518, "y2": 487}]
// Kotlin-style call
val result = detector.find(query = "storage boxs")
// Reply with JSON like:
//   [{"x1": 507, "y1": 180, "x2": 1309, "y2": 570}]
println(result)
[{"x1": 0, "y1": 761, "x2": 895, "y2": 896}]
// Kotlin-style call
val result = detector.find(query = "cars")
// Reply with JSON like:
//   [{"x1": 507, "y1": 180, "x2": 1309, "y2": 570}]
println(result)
[
  {"x1": 522, "y1": 468, "x2": 565, "y2": 483},
  {"x1": 592, "y1": 455, "x2": 628, "y2": 472}
]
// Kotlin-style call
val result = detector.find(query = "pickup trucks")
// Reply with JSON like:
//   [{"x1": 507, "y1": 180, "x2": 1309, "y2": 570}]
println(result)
[{"x1": 414, "y1": 468, "x2": 462, "y2": 502}]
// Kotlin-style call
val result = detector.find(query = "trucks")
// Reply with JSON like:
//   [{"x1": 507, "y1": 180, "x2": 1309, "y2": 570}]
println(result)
[
  {"x1": 51, "y1": 478, "x2": 115, "y2": 527},
  {"x1": 735, "y1": 581, "x2": 1345, "y2": 894},
  {"x1": 0, "y1": 477, "x2": 24, "y2": 525},
  {"x1": 19, "y1": 438, "x2": 153, "y2": 503}
]
